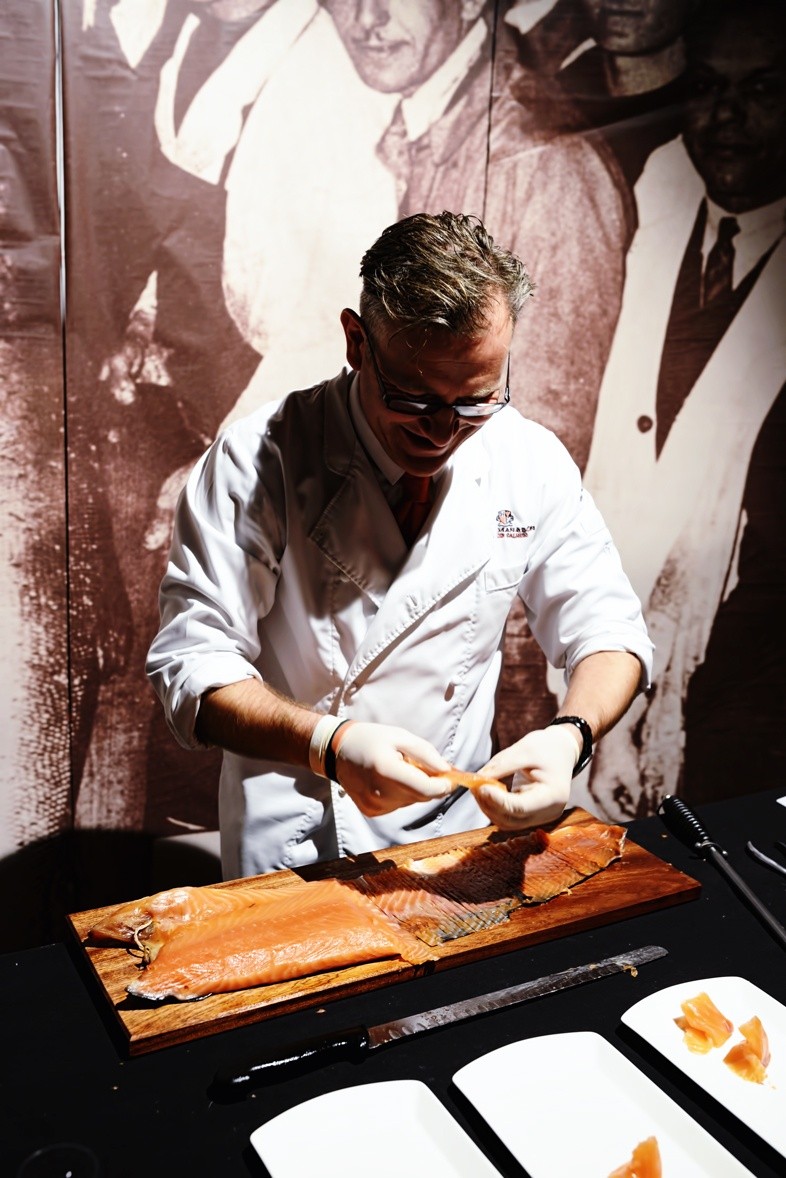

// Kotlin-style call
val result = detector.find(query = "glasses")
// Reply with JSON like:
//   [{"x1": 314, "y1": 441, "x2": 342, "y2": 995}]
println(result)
[{"x1": 366, "y1": 333, "x2": 511, "y2": 417}]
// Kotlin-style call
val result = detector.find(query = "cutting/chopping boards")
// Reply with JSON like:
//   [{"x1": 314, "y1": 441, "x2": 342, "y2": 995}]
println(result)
[{"x1": 67, "y1": 806, "x2": 704, "y2": 1056}]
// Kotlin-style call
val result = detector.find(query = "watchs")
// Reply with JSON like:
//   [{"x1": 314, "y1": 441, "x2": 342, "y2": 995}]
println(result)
[{"x1": 549, "y1": 716, "x2": 593, "y2": 779}]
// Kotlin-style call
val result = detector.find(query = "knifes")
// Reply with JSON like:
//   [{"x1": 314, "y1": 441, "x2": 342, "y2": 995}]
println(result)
[{"x1": 209, "y1": 945, "x2": 670, "y2": 1100}]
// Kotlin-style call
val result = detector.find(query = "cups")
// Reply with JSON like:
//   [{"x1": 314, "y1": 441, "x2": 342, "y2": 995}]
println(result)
[{"x1": 16, "y1": 1143, "x2": 103, "y2": 1177}]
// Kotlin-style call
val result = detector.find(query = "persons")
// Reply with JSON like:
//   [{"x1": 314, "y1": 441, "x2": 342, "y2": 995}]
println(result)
[
  {"x1": 0, "y1": 0, "x2": 786, "y2": 823},
  {"x1": 145, "y1": 211, "x2": 655, "y2": 884}
]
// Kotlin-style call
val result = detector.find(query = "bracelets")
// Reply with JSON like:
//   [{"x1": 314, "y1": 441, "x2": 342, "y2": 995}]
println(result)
[
  {"x1": 324, "y1": 719, "x2": 357, "y2": 785},
  {"x1": 119, "y1": 335, "x2": 145, "y2": 379}
]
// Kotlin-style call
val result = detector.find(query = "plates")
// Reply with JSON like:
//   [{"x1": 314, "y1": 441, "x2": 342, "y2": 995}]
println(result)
[
  {"x1": 452, "y1": 1031, "x2": 762, "y2": 1178},
  {"x1": 619, "y1": 975, "x2": 786, "y2": 1160},
  {"x1": 249, "y1": 1079, "x2": 505, "y2": 1178}
]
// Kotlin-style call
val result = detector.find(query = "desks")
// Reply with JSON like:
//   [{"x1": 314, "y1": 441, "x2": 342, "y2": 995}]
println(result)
[{"x1": 0, "y1": 786, "x2": 786, "y2": 1178}]
